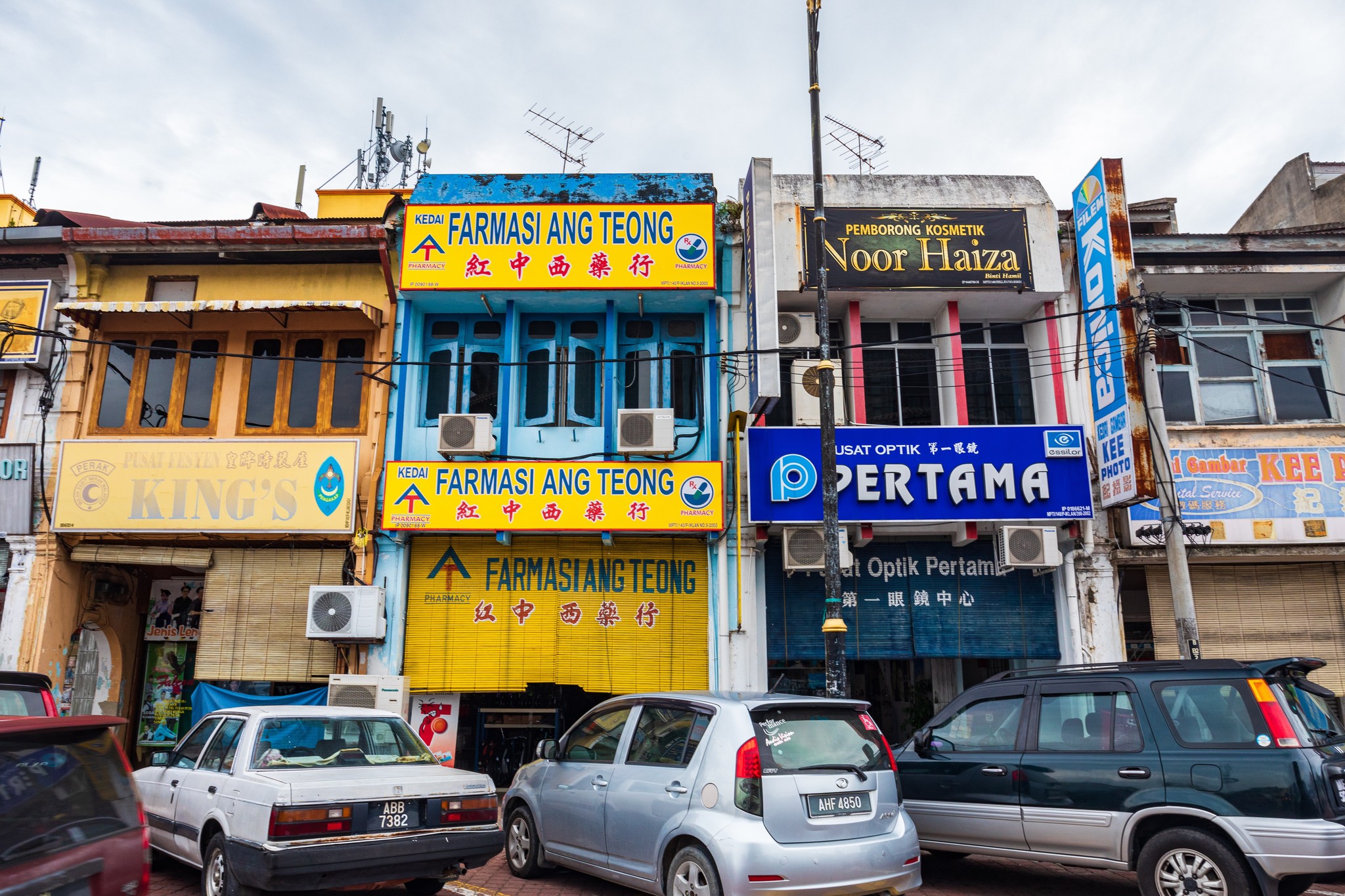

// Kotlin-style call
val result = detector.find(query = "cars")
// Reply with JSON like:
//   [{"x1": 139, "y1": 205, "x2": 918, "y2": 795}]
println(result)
[
  {"x1": 0, "y1": 712, "x2": 153, "y2": 896},
  {"x1": 128, "y1": 705, "x2": 507, "y2": 896},
  {"x1": 499, "y1": 690, "x2": 926, "y2": 896}
]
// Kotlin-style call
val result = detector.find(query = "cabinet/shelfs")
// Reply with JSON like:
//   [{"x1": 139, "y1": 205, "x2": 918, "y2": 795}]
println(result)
[{"x1": 475, "y1": 708, "x2": 564, "y2": 774}]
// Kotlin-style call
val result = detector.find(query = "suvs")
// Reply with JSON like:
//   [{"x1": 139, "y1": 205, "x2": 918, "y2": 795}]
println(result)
[
  {"x1": 0, "y1": 669, "x2": 61, "y2": 717},
  {"x1": 887, "y1": 654, "x2": 1345, "y2": 896}
]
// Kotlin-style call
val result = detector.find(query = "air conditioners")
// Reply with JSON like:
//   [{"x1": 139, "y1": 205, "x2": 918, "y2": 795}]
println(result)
[
  {"x1": 783, "y1": 526, "x2": 853, "y2": 571},
  {"x1": 778, "y1": 312, "x2": 820, "y2": 352},
  {"x1": 306, "y1": 584, "x2": 387, "y2": 643},
  {"x1": 617, "y1": 407, "x2": 677, "y2": 454},
  {"x1": 790, "y1": 358, "x2": 845, "y2": 427},
  {"x1": 325, "y1": 672, "x2": 411, "y2": 721},
  {"x1": 997, "y1": 525, "x2": 1064, "y2": 570},
  {"x1": 437, "y1": 412, "x2": 497, "y2": 456}
]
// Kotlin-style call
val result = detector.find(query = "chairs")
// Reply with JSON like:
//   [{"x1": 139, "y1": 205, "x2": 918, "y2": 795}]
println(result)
[
  {"x1": 1085, "y1": 713, "x2": 1109, "y2": 739},
  {"x1": 1060, "y1": 718, "x2": 1086, "y2": 745},
  {"x1": 256, "y1": 740, "x2": 273, "y2": 760},
  {"x1": 316, "y1": 739, "x2": 345, "y2": 758}
]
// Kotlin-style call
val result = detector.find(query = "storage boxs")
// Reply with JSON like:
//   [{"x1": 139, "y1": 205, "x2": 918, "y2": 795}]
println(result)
[{"x1": 504, "y1": 715, "x2": 543, "y2": 724}]
